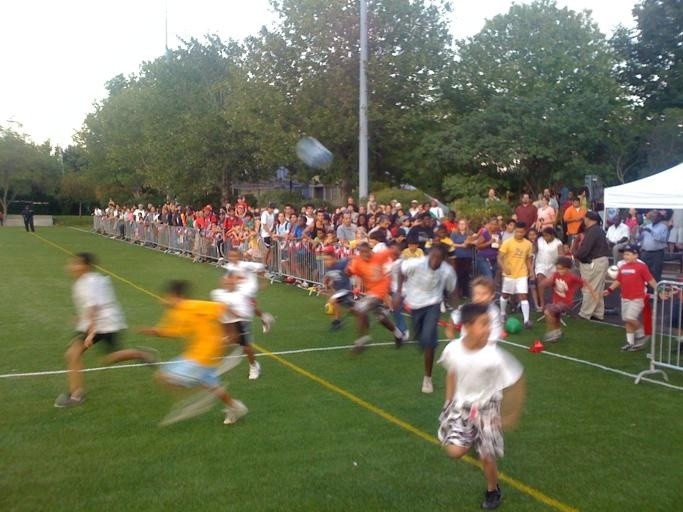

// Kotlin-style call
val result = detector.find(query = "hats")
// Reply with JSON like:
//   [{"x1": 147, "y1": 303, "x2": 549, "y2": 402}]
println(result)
[{"x1": 618, "y1": 245, "x2": 636, "y2": 254}]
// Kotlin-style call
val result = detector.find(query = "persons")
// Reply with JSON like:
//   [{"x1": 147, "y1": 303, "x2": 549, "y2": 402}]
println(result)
[
  {"x1": 0, "y1": 207, "x2": 4, "y2": 225},
  {"x1": 21, "y1": 202, "x2": 35, "y2": 232}
]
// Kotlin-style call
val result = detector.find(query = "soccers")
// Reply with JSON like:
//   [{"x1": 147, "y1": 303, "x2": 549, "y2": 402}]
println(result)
[
  {"x1": 506, "y1": 318, "x2": 522, "y2": 334},
  {"x1": 607, "y1": 264, "x2": 619, "y2": 280}
]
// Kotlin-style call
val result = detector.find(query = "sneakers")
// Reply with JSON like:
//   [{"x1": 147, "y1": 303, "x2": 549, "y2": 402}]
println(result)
[
  {"x1": 247, "y1": 360, "x2": 260, "y2": 380},
  {"x1": 54, "y1": 393, "x2": 85, "y2": 409},
  {"x1": 421, "y1": 377, "x2": 437, "y2": 394},
  {"x1": 94, "y1": 229, "x2": 225, "y2": 268},
  {"x1": 329, "y1": 318, "x2": 410, "y2": 353},
  {"x1": 483, "y1": 485, "x2": 501, "y2": 510},
  {"x1": 499, "y1": 305, "x2": 651, "y2": 351},
  {"x1": 224, "y1": 400, "x2": 248, "y2": 424},
  {"x1": 261, "y1": 314, "x2": 275, "y2": 333},
  {"x1": 265, "y1": 272, "x2": 327, "y2": 295}
]
